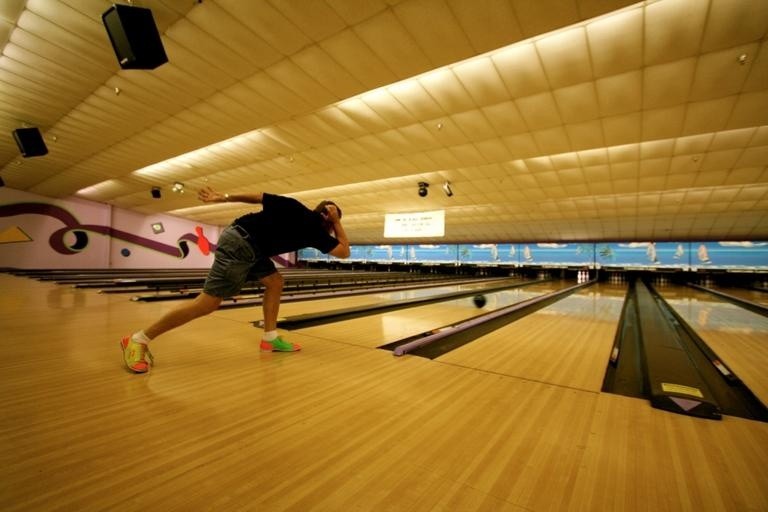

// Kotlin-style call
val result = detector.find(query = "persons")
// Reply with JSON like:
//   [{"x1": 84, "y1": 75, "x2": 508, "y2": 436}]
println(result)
[{"x1": 119, "y1": 184, "x2": 350, "y2": 373}]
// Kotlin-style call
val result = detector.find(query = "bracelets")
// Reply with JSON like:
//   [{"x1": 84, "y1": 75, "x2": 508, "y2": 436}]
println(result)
[{"x1": 224, "y1": 193, "x2": 232, "y2": 202}]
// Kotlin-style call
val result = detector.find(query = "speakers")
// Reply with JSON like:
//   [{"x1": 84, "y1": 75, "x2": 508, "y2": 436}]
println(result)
[
  {"x1": 12, "y1": 127, "x2": 49, "y2": 158},
  {"x1": 101, "y1": 4, "x2": 169, "y2": 70},
  {"x1": 151, "y1": 189, "x2": 161, "y2": 198}
]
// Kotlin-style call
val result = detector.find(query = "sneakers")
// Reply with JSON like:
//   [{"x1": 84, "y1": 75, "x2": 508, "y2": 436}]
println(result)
[
  {"x1": 120, "y1": 333, "x2": 149, "y2": 373},
  {"x1": 259, "y1": 336, "x2": 302, "y2": 352}
]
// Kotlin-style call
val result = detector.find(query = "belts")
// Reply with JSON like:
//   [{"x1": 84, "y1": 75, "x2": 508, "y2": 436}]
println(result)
[{"x1": 232, "y1": 223, "x2": 254, "y2": 245}]
